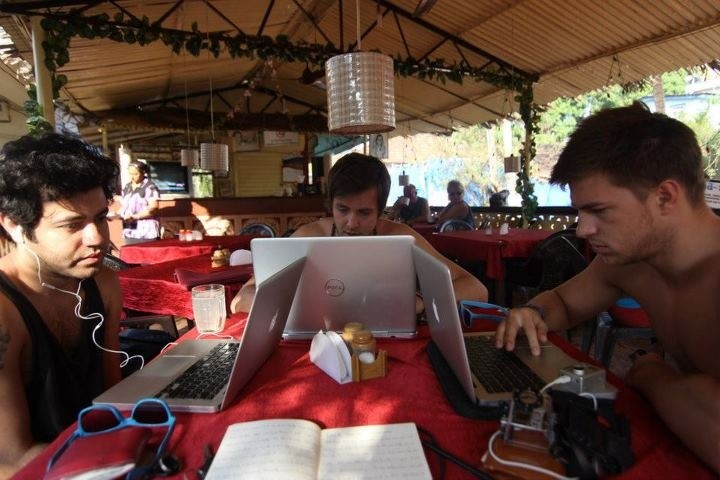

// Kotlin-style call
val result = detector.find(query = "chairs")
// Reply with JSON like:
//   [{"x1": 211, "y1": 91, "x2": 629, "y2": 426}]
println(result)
[
  {"x1": 503, "y1": 228, "x2": 585, "y2": 308},
  {"x1": 238, "y1": 223, "x2": 276, "y2": 238},
  {"x1": 440, "y1": 219, "x2": 474, "y2": 232}
]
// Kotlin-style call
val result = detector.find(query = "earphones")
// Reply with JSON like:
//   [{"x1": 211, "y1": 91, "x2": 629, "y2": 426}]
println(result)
[{"x1": 16, "y1": 224, "x2": 24, "y2": 245}]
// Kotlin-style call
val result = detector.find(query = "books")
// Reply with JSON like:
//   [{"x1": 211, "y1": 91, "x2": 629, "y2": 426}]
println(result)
[{"x1": 204, "y1": 418, "x2": 433, "y2": 480}]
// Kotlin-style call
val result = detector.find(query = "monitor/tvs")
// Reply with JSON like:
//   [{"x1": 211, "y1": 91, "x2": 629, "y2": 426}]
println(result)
[{"x1": 147, "y1": 159, "x2": 193, "y2": 200}]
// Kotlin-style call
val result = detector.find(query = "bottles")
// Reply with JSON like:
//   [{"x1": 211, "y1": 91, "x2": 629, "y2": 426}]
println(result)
[
  {"x1": 486, "y1": 223, "x2": 493, "y2": 235},
  {"x1": 342, "y1": 323, "x2": 364, "y2": 355},
  {"x1": 186, "y1": 229, "x2": 193, "y2": 244},
  {"x1": 179, "y1": 229, "x2": 186, "y2": 244},
  {"x1": 352, "y1": 331, "x2": 377, "y2": 363}
]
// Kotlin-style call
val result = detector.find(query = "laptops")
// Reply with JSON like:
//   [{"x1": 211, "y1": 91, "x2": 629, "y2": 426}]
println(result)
[
  {"x1": 410, "y1": 244, "x2": 622, "y2": 421},
  {"x1": 249, "y1": 233, "x2": 419, "y2": 341},
  {"x1": 92, "y1": 256, "x2": 308, "y2": 415}
]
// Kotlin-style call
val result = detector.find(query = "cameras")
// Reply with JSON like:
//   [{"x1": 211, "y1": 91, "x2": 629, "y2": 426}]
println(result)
[{"x1": 558, "y1": 361, "x2": 606, "y2": 384}]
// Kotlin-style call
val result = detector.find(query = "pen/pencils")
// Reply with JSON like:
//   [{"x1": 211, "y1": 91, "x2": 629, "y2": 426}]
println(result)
[{"x1": 422, "y1": 439, "x2": 495, "y2": 480}]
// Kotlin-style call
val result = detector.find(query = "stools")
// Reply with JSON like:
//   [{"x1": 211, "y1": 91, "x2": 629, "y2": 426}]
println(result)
[{"x1": 581, "y1": 311, "x2": 654, "y2": 368}]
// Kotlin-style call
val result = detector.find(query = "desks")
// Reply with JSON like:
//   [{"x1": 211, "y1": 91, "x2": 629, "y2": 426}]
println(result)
[{"x1": 9, "y1": 223, "x2": 719, "y2": 480}]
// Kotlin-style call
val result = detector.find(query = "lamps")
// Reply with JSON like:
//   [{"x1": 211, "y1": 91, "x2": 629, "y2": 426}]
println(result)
[
  {"x1": 181, "y1": 0, "x2": 228, "y2": 171},
  {"x1": 399, "y1": 170, "x2": 408, "y2": 185},
  {"x1": 504, "y1": 154, "x2": 520, "y2": 173},
  {"x1": 325, "y1": 0, "x2": 396, "y2": 134}
]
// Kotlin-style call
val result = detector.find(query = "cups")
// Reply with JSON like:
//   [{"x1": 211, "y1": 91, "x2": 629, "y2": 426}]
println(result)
[
  {"x1": 402, "y1": 197, "x2": 410, "y2": 208},
  {"x1": 191, "y1": 284, "x2": 226, "y2": 334}
]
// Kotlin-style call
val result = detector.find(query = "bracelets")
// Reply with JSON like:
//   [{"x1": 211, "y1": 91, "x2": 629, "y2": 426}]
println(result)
[
  {"x1": 416, "y1": 292, "x2": 425, "y2": 315},
  {"x1": 515, "y1": 303, "x2": 545, "y2": 320}
]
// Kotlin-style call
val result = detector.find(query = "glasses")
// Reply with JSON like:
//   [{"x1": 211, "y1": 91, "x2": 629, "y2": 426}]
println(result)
[
  {"x1": 449, "y1": 191, "x2": 464, "y2": 196},
  {"x1": 47, "y1": 398, "x2": 176, "y2": 479}
]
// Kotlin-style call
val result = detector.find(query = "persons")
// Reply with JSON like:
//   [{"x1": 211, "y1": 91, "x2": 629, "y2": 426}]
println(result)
[
  {"x1": 230, "y1": 152, "x2": 489, "y2": 316},
  {"x1": 494, "y1": 100, "x2": 720, "y2": 476},
  {"x1": 386, "y1": 180, "x2": 475, "y2": 232},
  {"x1": 0, "y1": 124, "x2": 124, "y2": 480},
  {"x1": 113, "y1": 161, "x2": 160, "y2": 220}
]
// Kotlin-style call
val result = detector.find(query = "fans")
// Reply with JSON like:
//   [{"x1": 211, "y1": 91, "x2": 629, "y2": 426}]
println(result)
[{"x1": 278, "y1": 61, "x2": 325, "y2": 92}]
